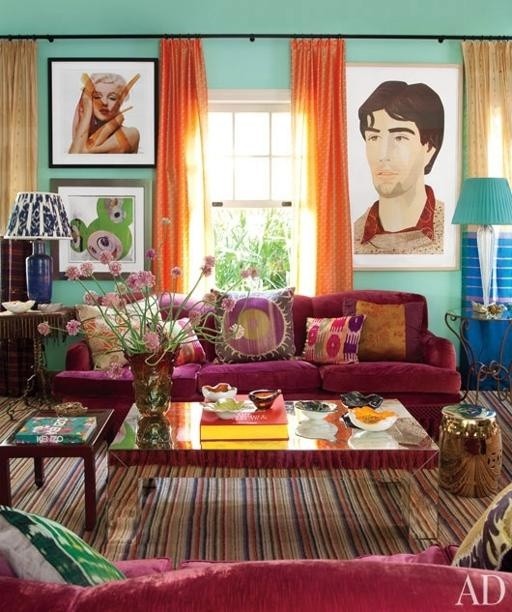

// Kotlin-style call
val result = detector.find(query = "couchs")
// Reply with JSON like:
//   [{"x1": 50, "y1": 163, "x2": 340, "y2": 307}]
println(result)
[
  {"x1": 51, "y1": 291, "x2": 462, "y2": 440},
  {"x1": 0, "y1": 554, "x2": 512, "y2": 612}
]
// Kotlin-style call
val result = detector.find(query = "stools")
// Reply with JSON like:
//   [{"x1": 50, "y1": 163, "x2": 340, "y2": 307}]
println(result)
[{"x1": 438, "y1": 402, "x2": 502, "y2": 497}]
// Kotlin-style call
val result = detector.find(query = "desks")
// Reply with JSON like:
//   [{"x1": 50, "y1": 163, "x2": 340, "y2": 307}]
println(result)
[{"x1": 0, "y1": 409, "x2": 117, "y2": 532}]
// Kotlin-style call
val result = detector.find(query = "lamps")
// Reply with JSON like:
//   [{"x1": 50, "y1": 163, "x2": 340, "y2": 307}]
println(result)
[
  {"x1": 3, "y1": 191, "x2": 73, "y2": 302},
  {"x1": 451, "y1": 176, "x2": 512, "y2": 314}
]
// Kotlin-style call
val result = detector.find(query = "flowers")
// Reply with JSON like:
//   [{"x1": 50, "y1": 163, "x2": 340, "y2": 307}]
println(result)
[{"x1": 35, "y1": 248, "x2": 258, "y2": 353}]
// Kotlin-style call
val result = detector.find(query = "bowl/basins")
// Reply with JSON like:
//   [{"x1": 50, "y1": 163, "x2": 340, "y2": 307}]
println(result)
[
  {"x1": 201, "y1": 382, "x2": 400, "y2": 432},
  {"x1": 1, "y1": 299, "x2": 36, "y2": 314}
]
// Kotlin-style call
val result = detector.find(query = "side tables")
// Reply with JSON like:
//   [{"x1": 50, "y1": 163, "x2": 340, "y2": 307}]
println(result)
[
  {"x1": 444, "y1": 306, "x2": 512, "y2": 406},
  {"x1": 0, "y1": 307, "x2": 74, "y2": 422}
]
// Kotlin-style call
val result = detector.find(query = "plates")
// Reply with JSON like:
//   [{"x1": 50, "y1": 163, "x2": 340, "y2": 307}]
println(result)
[{"x1": 37, "y1": 303, "x2": 64, "y2": 313}]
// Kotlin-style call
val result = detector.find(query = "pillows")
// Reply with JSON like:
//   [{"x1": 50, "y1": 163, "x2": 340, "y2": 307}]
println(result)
[
  {"x1": 342, "y1": 297, "x2": 426, "y2": 361},
  {"x1": 300, "y1": 314, "x2": 367, "y2": 366},
  {"x1": 150, "y1": 317, "x2": 207, "y2": 366},
  {"x1": 74, "y1": 295, "x2": 162, "y2": 369},
  {"x1": 450, "y1": 478, "x2": 512, "y2": 571},
  {"x1": 207, "y1": 285, "x2": 301, "y2": 362},
  {"x1": 0, "y1": 504, "x2": 128, "y2": 590}
]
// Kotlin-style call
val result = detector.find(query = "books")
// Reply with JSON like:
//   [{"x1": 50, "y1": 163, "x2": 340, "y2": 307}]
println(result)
[
  {"x1": 199, "y1": 393, "x2": 290, "y2": 441},
  {"x1": 14, "y1": 415, "x2": 97, "y2": 445}
]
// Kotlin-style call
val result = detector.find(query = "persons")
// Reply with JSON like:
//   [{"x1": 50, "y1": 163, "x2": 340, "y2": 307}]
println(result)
[
  {"x1": 67, "y1": 71, "x2": 140, "y2": 155},
  {"x1": 352, "y1": 80, "x2": 446, "y2": 256}
]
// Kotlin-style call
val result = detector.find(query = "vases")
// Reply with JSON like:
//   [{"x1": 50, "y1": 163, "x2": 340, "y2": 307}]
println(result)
[{"x1": 119, "y1": 352, "x2": 176, "y2": 451}]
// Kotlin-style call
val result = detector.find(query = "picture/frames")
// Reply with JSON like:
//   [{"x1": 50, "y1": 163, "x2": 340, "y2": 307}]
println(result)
[
  {"x1": 341, "y1": 60, "x2": 465, "y2": 273},
  {"x1": 47, "y1": 57, "x2": 159, "y2": 170},
  {"x1": 47, "y1": 178, "x2": 157, "y2": 282}
]
